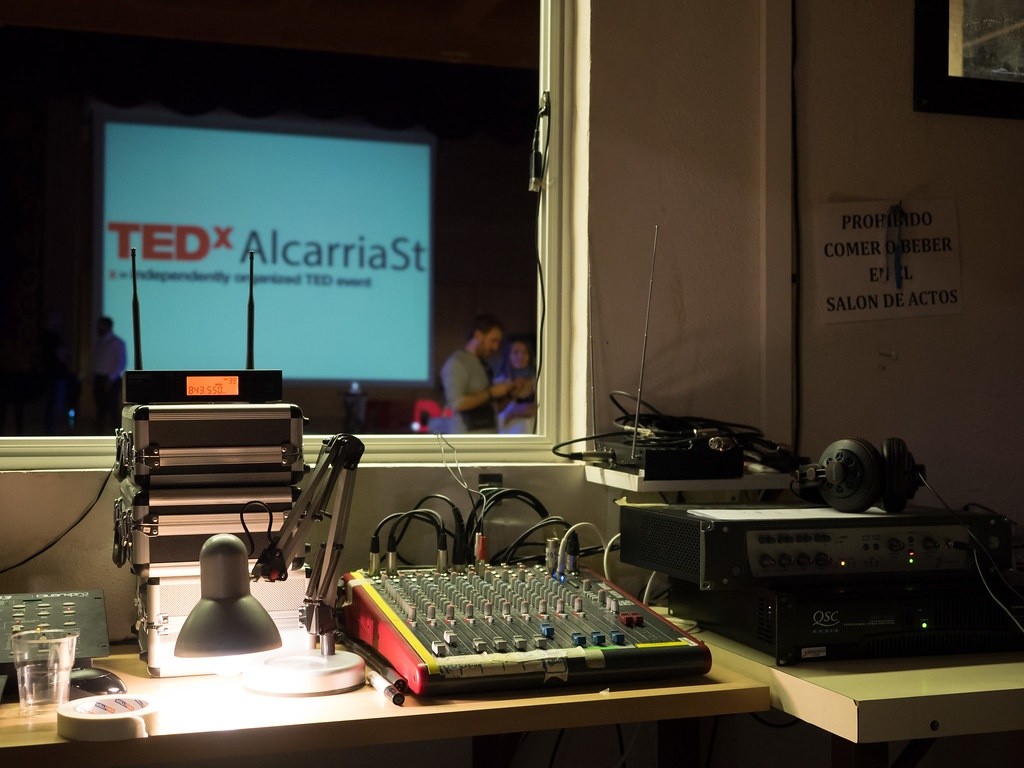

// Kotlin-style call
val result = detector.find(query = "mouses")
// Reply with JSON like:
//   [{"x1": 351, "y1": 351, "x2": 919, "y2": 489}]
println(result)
[{"x1": 68, "y1": 668, "x2": 128, "y2": 702}]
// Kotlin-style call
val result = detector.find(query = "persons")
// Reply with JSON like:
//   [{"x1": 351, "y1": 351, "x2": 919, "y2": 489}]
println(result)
[
  {"x1": 441, "y1": 320, "x2": 537, "y2": 434},
  {"x1": 36, "y1": 316, "x2": 125, "y2": 436}
]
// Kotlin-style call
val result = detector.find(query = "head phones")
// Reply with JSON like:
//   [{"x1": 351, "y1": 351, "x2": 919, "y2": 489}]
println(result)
[{"x1": 792, "y1": 436, "x2": 927, "y2": 514}]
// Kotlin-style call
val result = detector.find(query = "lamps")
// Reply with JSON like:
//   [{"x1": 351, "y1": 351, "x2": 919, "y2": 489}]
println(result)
[{"x1": 171, "y1": 433, "x2": 367, "y2": 698}]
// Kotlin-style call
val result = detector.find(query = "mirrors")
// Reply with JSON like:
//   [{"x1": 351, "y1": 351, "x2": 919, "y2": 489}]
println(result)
[{"x1": 913, "y1": 0, "x2": 1024, "y2": 121}]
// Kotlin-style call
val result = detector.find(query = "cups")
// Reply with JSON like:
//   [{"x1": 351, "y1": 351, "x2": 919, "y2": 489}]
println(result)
[{"x1": 11, "y1": 629, "x2": 79, "y2": 715}]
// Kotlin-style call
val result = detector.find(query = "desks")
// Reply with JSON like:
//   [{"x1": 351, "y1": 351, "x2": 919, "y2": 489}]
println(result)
[{"x1": 0, "y1": 607, "x2": 1024, "y2": 768}]
